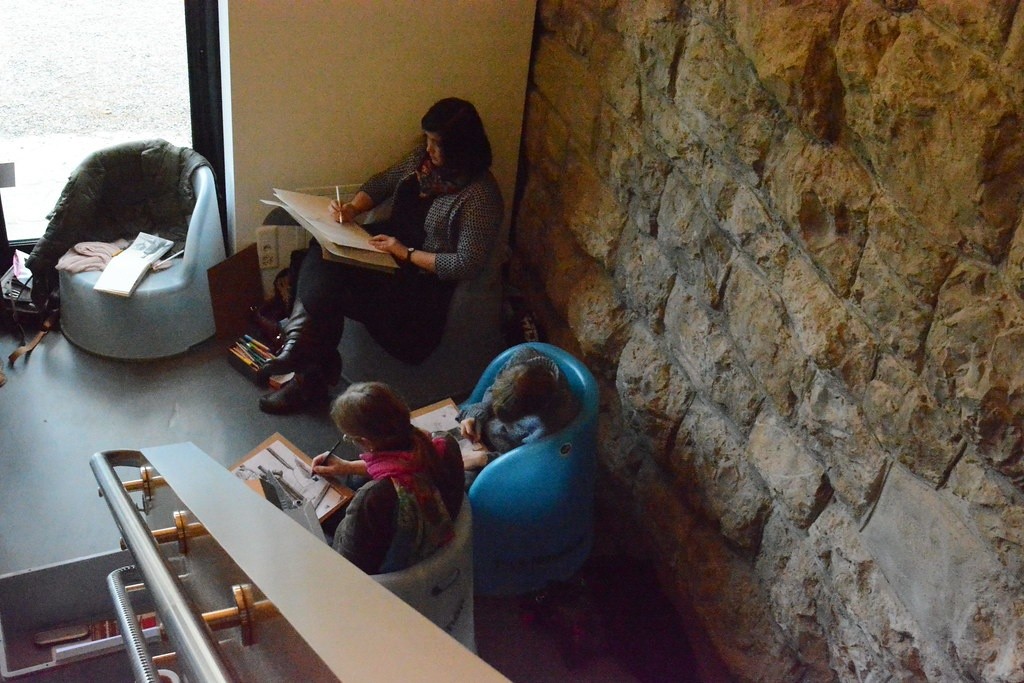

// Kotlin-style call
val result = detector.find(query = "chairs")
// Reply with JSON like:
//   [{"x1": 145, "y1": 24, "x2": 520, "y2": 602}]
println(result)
[
  {"x1": 55, "y1": 147, "x2": 227, "y2": 362},
  {"x1": 457, "y1": 342, "x2": 601, "y2": 598},
  {"x1": 335, "y1": 238, "x2": 509, "y2": 410},
  {"x1": 367, "y1": 490, "x2": 478, "y2": 657}
]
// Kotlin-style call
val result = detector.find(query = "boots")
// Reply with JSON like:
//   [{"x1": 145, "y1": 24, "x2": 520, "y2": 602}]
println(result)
[
  {"x1": 262, "y1": 332, "x2": 322, "y2": 376},
  {"x1": 260, "y1": 377, "x2": 329, "y2": 414}
]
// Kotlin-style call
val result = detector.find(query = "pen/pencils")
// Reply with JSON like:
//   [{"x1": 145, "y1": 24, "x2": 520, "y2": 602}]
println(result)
[
  {"x1": 230, "y1": 334, "x2": 279, "y2": 371},
  {"x1": 335, "y1": 185, "x2": 343, "y2": 225},
  {"x1": 470, "y1": 414, "x2": 477, "y2": 444},
  {"x1": 309, "y1": 440, "x2": 341, "y2": 477}
]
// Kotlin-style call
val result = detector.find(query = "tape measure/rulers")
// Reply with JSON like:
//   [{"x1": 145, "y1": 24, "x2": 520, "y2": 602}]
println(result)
[{"x1": 312, "y1": 482, "x2": 330, "y2": 510}]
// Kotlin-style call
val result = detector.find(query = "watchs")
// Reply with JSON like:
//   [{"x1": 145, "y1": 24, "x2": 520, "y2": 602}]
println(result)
[{"x1": 407, "y1": 246, "x2": 415, "y2": 263}]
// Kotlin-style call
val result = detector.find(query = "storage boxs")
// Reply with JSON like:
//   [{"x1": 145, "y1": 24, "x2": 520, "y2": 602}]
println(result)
[{"x1": 227, "y1": 342, "x2": 271, "y2": 386}]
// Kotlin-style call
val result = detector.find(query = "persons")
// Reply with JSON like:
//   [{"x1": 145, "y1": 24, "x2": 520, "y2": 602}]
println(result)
[
  {"x1": 310, "y1": 380, "x2": 465, "y2": 569},
  {"x1": 456, "y1": 349, "x2": 582, "y2": 473},
  {"x1": 256, "y1": 93, "x2": 507, "y2": 415}
]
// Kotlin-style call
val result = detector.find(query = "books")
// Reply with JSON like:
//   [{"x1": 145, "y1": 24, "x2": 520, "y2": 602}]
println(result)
[{"x1": 93, "y1": 231, "x2": 175, "y2": 298}]
